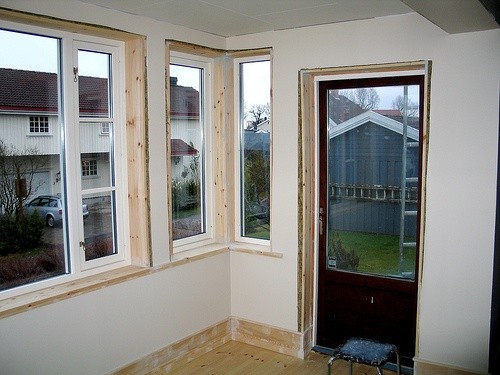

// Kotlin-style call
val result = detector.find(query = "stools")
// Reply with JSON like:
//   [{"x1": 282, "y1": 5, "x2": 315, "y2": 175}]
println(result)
[{"x1": 327, "y1": 337, "x2": 401, "y2": 375}]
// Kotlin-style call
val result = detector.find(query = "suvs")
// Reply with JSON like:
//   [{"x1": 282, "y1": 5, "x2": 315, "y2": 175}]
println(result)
[{"x1": 21, "y1": 193, "x2": 90, "y2": 227}]
